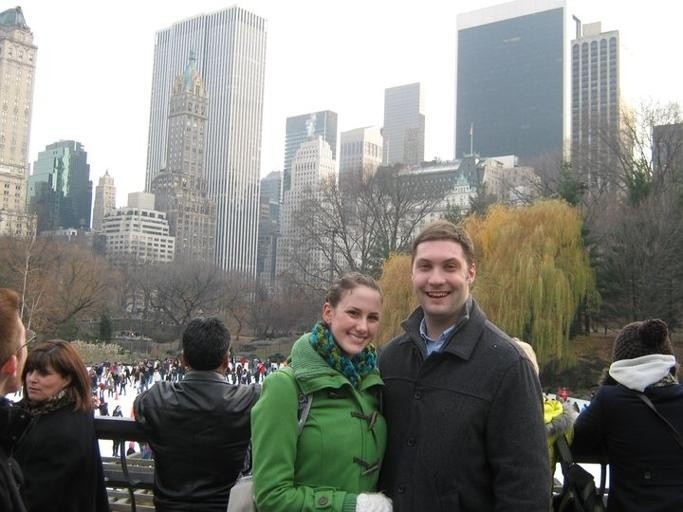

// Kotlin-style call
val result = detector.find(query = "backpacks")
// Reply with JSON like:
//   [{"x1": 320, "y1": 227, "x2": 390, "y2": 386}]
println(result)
[
  {"x1": 223, "y1": 362, "x2": 318, "y2": 512},
  {"x1": 551, "y1": 434, "x2": 608, "y2": 512}
]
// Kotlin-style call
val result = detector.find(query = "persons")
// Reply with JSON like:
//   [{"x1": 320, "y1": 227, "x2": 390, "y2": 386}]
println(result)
[
  {"x1": 378, "y1": 224, "x2": 553, "y2": 512},
  {"x1": 556, "y1": 385, "x2": 594, "y2": 414},
  {"x1": 251, "y1": 273, "x2": 393, "y2": 512},
  {"x1": 225, "y1": 358, "x2": 277, "y2": 384},
  {"x1": 569, "y1": 319, "x2": 683, "y2": 512},
  {"x1": 87, "y1": 358, "x2": 186, "y2": 496},
  {"x1": 133, "y1": 315, "x2": 261, "y2": 512},
  {"x1": 1, "y1": 288, "x2": 111, "y2": 512},
  {"x1": 512, "y1": 338, "x2": 577, "y2": 495}
]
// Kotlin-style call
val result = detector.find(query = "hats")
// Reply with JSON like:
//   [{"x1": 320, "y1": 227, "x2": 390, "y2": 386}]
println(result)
[{"x1": 613, "y1": 318, "x2": 675, "y2": 360}]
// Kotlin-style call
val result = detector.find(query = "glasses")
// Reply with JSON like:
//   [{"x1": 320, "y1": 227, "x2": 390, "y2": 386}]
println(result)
[{"x1": 0, "y1": 327, "x2": 36, "y2": 369}]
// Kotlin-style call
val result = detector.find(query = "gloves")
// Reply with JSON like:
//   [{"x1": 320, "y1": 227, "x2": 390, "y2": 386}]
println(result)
[{"x1": 356, "y1": 491, "x2": 393, "y2": 512}]
